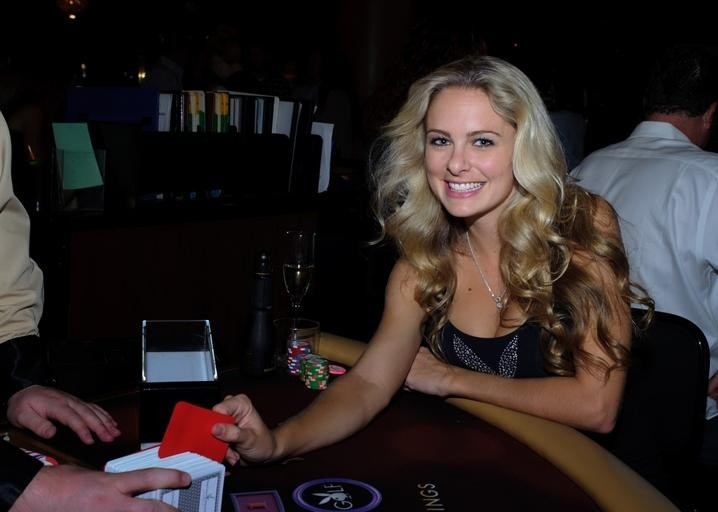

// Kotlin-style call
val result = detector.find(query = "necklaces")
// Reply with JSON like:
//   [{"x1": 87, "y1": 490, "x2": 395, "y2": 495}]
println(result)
[{"x1": 460, "y1": 224, "x2": 509, "y2": 312}]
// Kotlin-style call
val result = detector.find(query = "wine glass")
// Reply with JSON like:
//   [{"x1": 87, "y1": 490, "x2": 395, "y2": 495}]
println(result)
[{"x1": 276, "y1": 228, "x2": 319, "y2": 319}]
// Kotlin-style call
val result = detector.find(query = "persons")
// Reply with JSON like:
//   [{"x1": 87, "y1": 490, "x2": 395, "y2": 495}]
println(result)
[
  {"x1": 0, "y1": 104, "x2": 195, "y2": 512},
  {"x1": 566, "y1": 42, "x2": 717, "y2": 509},
  {"x1": 211, "y1": 52, "x2": 635, "y2": 468}
]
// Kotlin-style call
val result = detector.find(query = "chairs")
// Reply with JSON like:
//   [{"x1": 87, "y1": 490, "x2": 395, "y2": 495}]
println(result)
[{"x1": 576, "y1": 307, "x2": 710, "y2": 511}]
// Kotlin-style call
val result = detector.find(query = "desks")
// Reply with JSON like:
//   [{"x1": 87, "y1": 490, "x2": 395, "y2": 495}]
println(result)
[{"x1": 6, "y1": 330, "x2": 675, "y2": 510}]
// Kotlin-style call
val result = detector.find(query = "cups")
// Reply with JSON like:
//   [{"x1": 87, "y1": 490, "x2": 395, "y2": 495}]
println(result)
[{"x1": 279, "y1": 316, "x2": 321, "y2": 379}]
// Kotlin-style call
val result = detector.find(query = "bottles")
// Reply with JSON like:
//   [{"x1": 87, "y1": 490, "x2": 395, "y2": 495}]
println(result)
[{"x1": 232, "y1": 245, "x2": 288, "y2": 382}]
